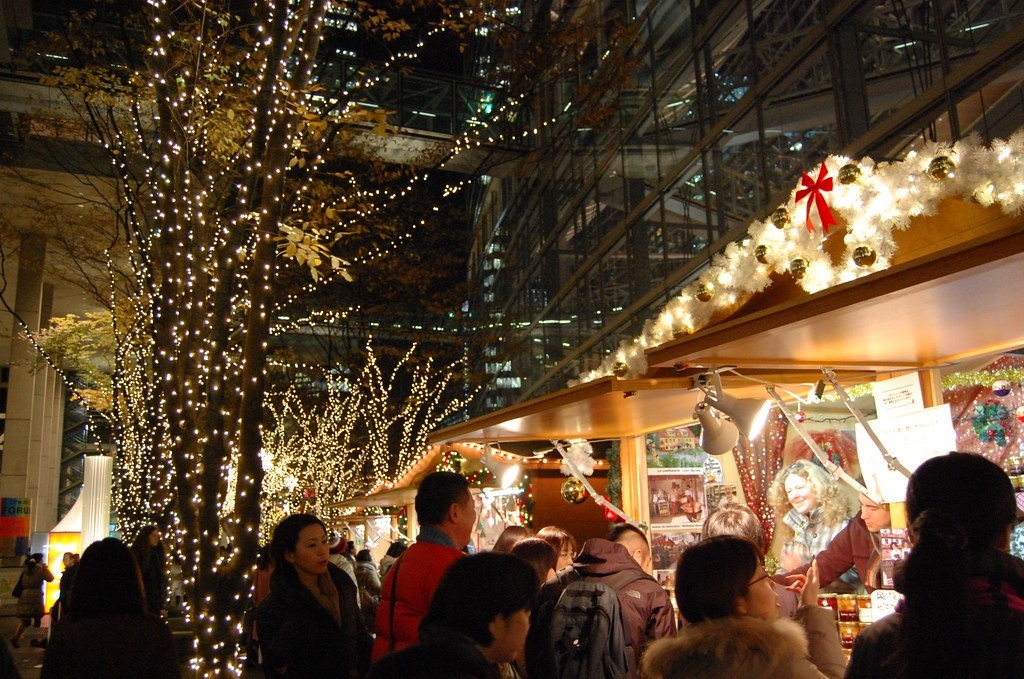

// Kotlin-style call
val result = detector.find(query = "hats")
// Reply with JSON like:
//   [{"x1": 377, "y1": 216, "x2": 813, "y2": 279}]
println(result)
[
  {"x1": 328, "y1": 536, "x2": 348, "y2": 554},
  {"x1": 906, "y1": 452, "x2": 1024, "y2": 534}
]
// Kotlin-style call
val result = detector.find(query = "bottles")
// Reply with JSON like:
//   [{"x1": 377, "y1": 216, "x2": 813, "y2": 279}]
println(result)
[
  {"x1": 658, "y1": 574, "x2": 671, "y2": 598},
  {"x1": 649, "y1": 482, "x2": 702, "y2": 522},
  {"x1": 652, "y1": 532, "x2": 700, "y2": 570}
]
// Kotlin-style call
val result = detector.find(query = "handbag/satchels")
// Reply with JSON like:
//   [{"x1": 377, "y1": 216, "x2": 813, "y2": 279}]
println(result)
[
  {"x1": 243, "y1": 596, "x2": 256, "y2": 633},
  {"x1": 12, "y1": 571, "x2": 24, "y2": 598}
]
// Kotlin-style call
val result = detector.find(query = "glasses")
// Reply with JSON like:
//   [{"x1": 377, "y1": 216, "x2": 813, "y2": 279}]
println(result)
[{"x1": 747, "y1": 566, "x2": 777, "y2": 588}]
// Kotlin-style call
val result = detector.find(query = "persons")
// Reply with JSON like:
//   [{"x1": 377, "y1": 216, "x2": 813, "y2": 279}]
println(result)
[
  {"x1": 39, "y1": 537, "x2": 182, "y2": 679},
  {"x1": 843, "y1": 451, "x2": 1024, "y2": 679},
  {"x1": 327, "y1": 533, "x2": 408, "y2": 611},
  {"x1": 644, "y1": 534, "x2": 847, "y2": 679},
  {"x1": 371, "y1": 551, "x2": 540, "y2": 679},
  {"x1": 131, "y1": 525, "x2": 169, "y2": 616},
  {"x1": 526, "y1": 524, "x2": 677, "y2": 679},
  {"x1": 702, "y1": 502, "x2": 797, "y2": 618},
  {"x1": 770, "y1": 458, "x2": 859, "y2": 594},
  {"x1": 60, "y1": 549, "x2": 78, "y2": 594},
  {"x1": 494, "y1": 526, "x2": 576, "y2": 585},
  {"x1": 11, "y1": 553, "x2": 54, "y2": 649},
  {"x1": 257, "y1": 513, "x2": 375, "y2": 679},
  {"x1": 772, "y1": 479, "x2": 890, "y2": 595},
  {"x1": 371, "y1": 471, "x2": 477, "y2": 664},
  {"x1": 252, "y1": 544, "x2": 278, "y2": 642}
]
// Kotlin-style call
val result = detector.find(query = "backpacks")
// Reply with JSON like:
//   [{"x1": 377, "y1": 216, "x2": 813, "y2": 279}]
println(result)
[{"x1": 545, "y1": 566, "x2": 658, "y2": 679}]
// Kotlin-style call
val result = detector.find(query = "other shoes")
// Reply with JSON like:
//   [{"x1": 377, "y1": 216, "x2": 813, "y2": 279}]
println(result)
[
  {"x1": 8, "y1": 635, "x2": 20, "y2": 648},
  {"x1": 30, "y1": 639, "x2": 41, "y2": 647}
]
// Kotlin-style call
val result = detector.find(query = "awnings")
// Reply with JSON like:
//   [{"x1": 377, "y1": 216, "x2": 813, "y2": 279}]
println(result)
[{"x1": 425, "y1": 129, "x2": 1024, "y2": 445}]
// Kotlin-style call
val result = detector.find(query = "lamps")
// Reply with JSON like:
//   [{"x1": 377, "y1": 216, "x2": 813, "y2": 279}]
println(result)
[
  {"x1": 481, "y1": 452, "x2": 519, "y2": 490},
  {"x1": 693, "y1": 365, "x2": 771, "y2": 455}
]
